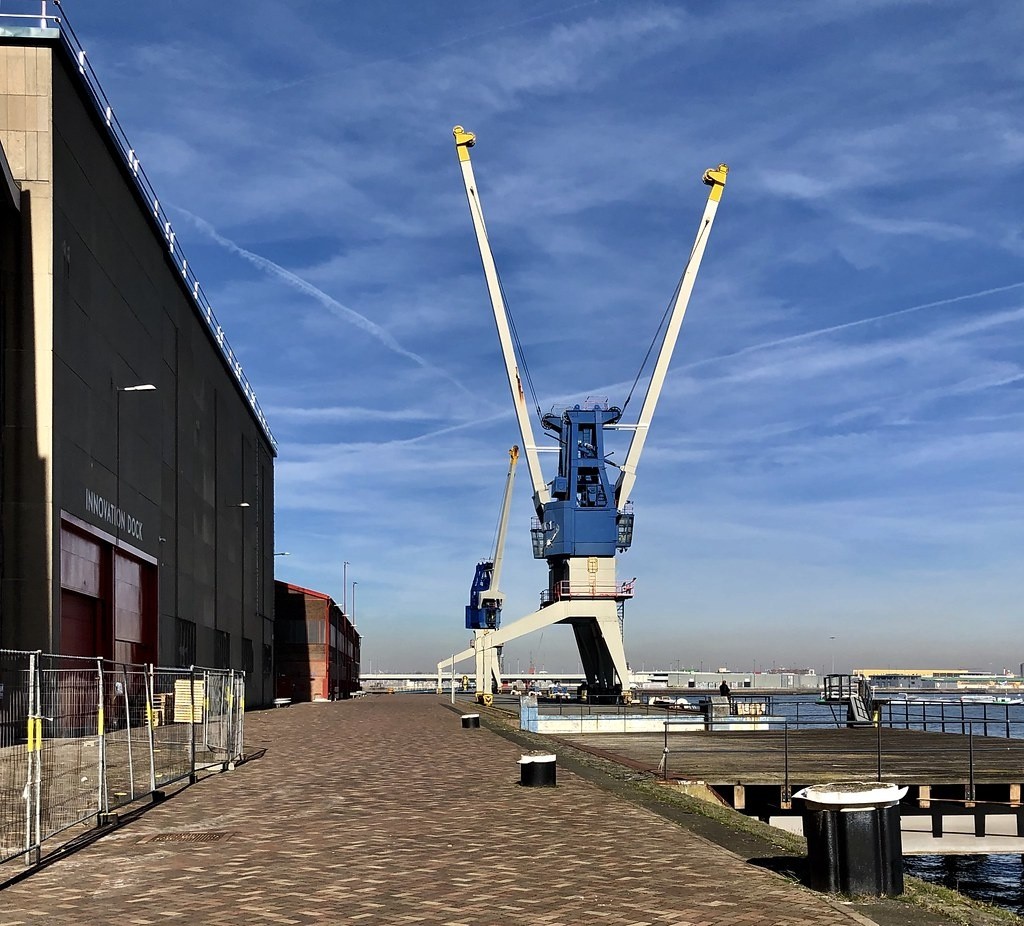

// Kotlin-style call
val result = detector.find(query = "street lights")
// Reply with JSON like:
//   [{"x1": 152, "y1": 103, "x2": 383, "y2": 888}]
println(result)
[
  {"x1": 830, "y1": 636, "x2": 836, "y2": 673},
  {"x1": 701, "y1": 660, "x2": 703, "y2": 673},
  {"x1": 343, "y1": 560, "x2": 351, "y2": 615},
  {"x1": 678, "y1": 659, "x2": 680, "y2": 672},
  {"x1": 753, "y1": 659, "x2": 755, "y2": 674},
  {"x1": 352, "y1": 581, "x2": 358, "y2": 625}
]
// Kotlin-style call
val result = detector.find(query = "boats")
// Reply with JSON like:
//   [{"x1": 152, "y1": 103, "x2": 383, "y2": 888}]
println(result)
[{"x1": 815, "y1": 687, "x2": 1024, "y2": 706}]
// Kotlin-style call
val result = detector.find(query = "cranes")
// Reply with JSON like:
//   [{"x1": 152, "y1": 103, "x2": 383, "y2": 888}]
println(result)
[{"x1": 437, "y1": 123, "x2": 729, "y2": 708}]
